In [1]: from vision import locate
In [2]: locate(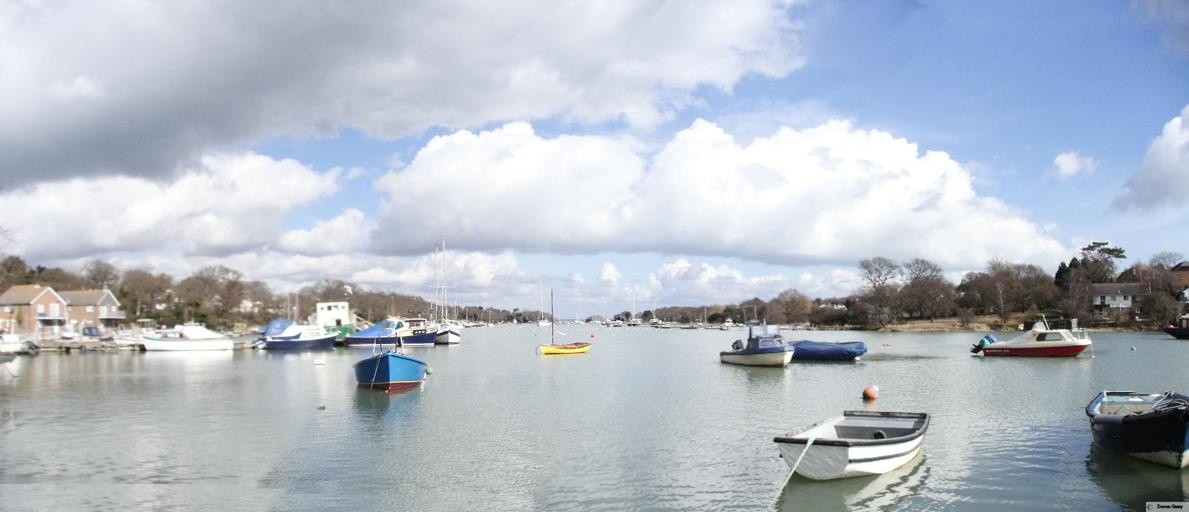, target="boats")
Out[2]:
[772,407,932,479]
[0,318,459,356]
[539,342,590,354]
[720,327,795,368]
[982,329,1092,357]
[463,318,735,331]
[788,340,866,361]
[1162,313,1189,338]
[1086,389,1188,471]
[352,352,430,392]
